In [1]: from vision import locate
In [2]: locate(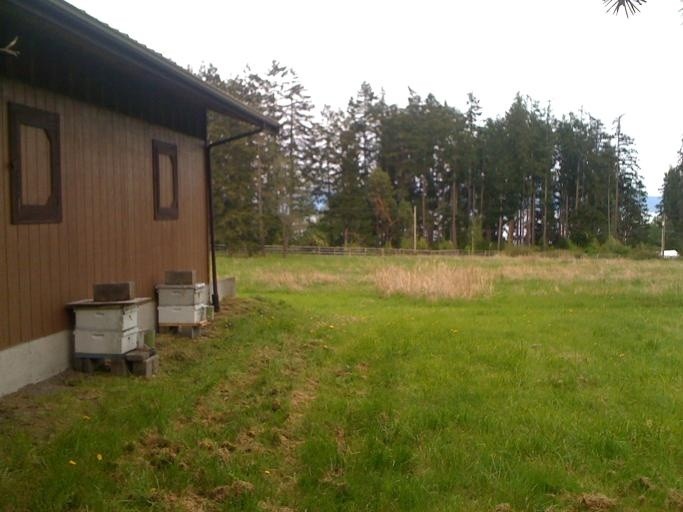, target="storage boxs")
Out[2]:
[73,304,137,330]
[158,304,206,322]
[73,327,137,353]
[154,281,207,304]
